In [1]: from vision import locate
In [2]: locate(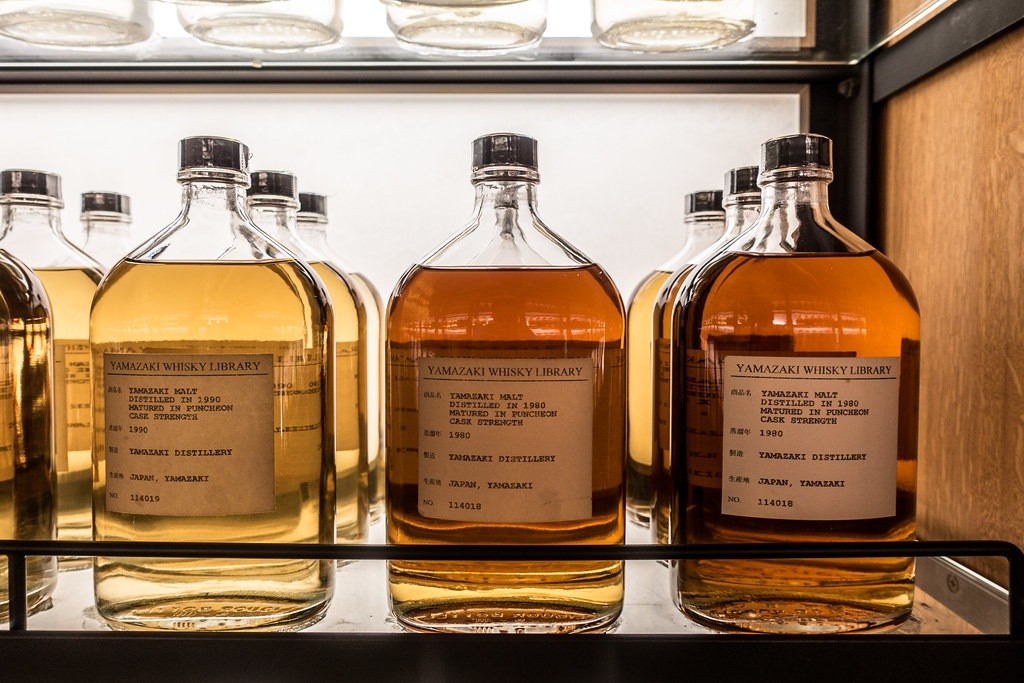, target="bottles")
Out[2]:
[0,135,922,634]
[0,0,757,58]
